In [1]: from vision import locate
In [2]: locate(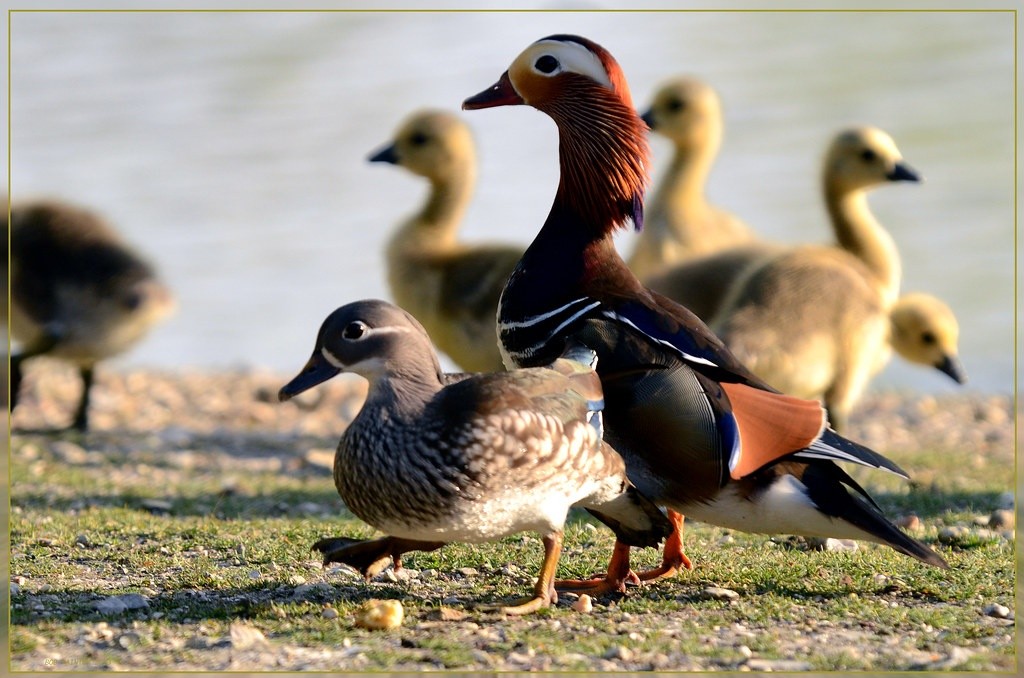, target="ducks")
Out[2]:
[646,128,924,326]
[277,300,636,615]
[367,106,531,377]
[464,35,950,597]
[1,196,170,433]
[623,74,760,285]
[704,242,964,441]
